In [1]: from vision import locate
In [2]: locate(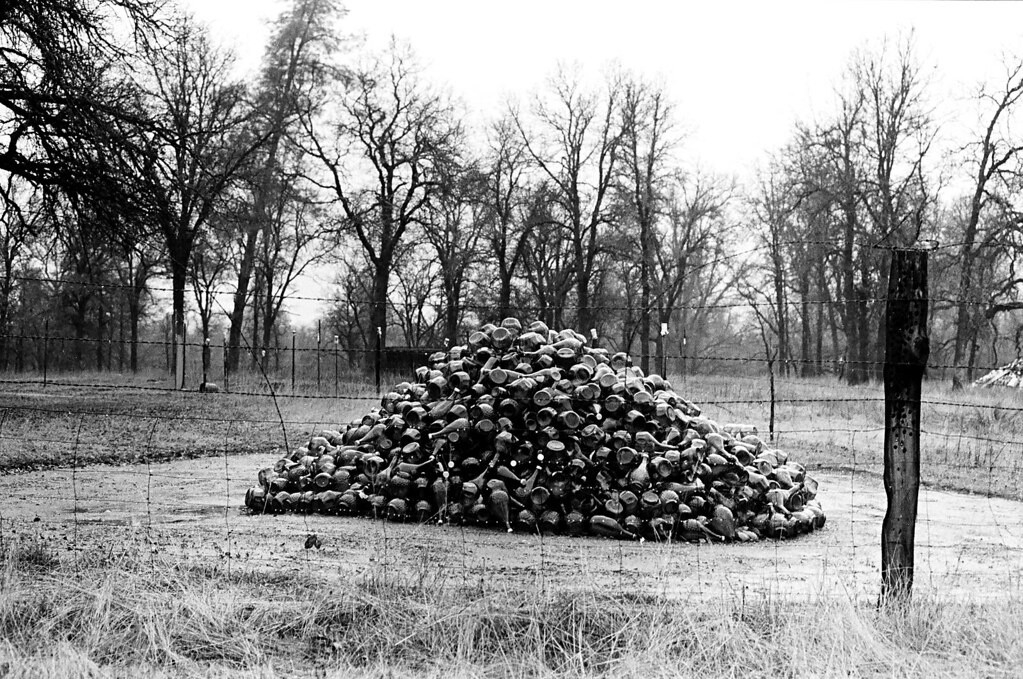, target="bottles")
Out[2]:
[243,318,825,539]
